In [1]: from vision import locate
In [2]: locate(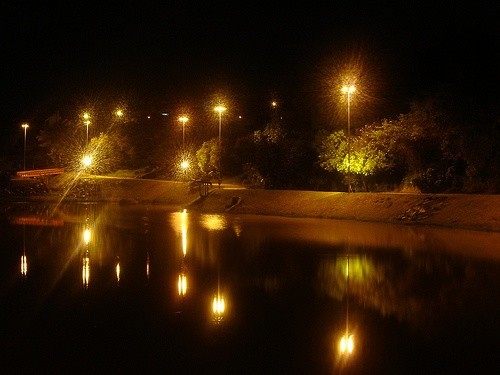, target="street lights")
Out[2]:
[80,214,91,290]
[211,231,228,322]
[212,106,227,188]
[142,206,154,280]
[174,113,190,184]
[77,115,94,181]
[338,255,358,359]
[339,84,359,193]
[19,223,30,279]
[19,122,30,172]
[176,209,194,299]
[114,221,125,285]
